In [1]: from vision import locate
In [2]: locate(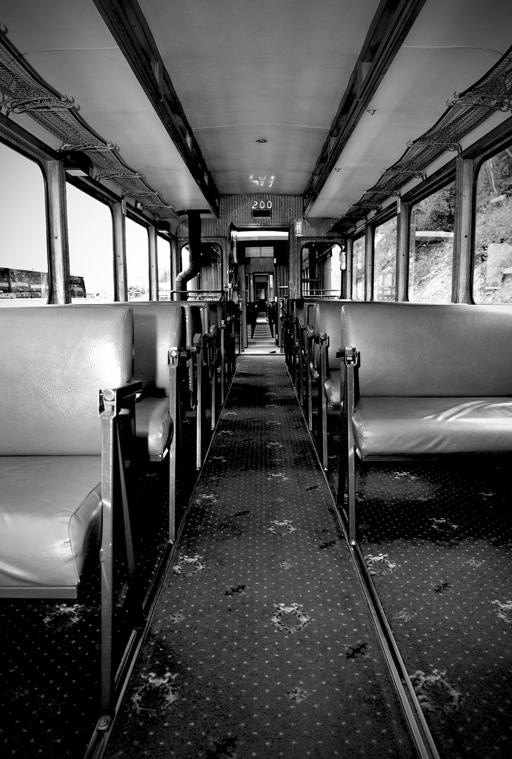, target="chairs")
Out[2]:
[0,305,145,731]
[208,301,227,406]
[189,302,217,430]
[303,302,315,431]
[226,301,236,385]
[310,303,469,472]
[336,304,512,547]
[284,298,352,407]
[131,302,203,471]
[133,304,191,545]
